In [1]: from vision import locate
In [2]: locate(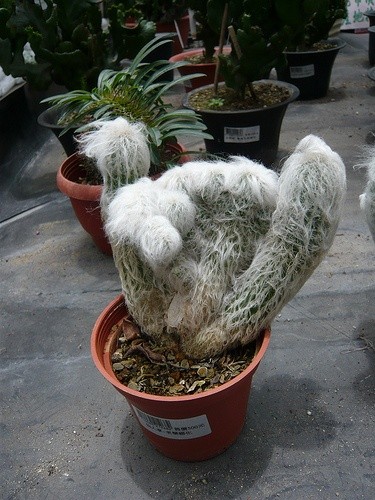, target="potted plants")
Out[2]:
[39,32,228,256]
[182,0,300,167]
[270,0,348,101]
[90,133,349,463]
[168,0,232,94]
[0,0,129,157]
